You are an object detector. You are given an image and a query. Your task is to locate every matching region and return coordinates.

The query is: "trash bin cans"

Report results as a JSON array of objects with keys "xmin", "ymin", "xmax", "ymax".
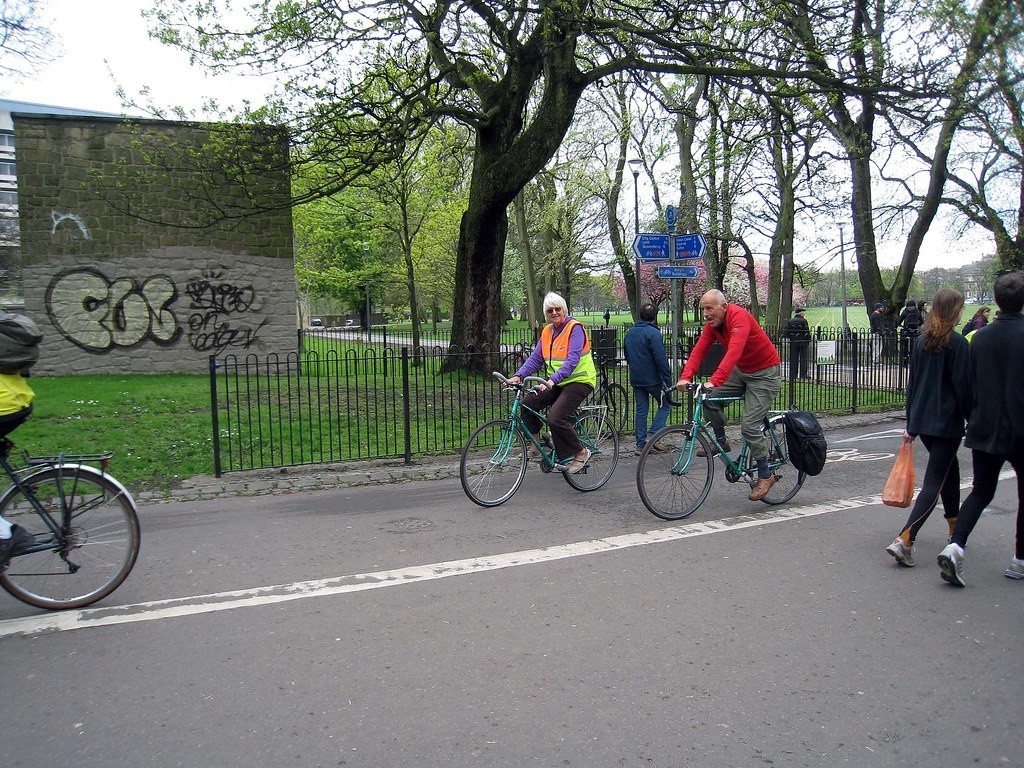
[
  {"xmin": 591, "ymin": 327, "xmax": 617, "ymax": 366},
  {"xmin": 700, "ymin": 341, "xmax": 724, "ymax": 378}
]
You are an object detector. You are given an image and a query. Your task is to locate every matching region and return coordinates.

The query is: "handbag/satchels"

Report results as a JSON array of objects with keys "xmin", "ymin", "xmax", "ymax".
[{"xmin": 883, "ymin": 441, "xmax": 914, "ymax": 507}]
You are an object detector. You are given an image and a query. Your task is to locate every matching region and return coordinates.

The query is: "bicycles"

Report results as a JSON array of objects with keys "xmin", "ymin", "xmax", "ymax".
[
  {"xmin": 636, "ymin": 381, "xmax": 810, "ymax": 523},
  {"xmin": 887, "ymin": 324, "xmax": 910, "ymax": 367},
  {"xmin": 0, "ymin": 434, "xmax": 140, "ymax": 611},
  {"xmin": 578, "ymin": 355, "xmax": 629, "ymax": 438},
  {"xmin": 460, "ymin": 370, "xmax": 622, "ymax": 509}
]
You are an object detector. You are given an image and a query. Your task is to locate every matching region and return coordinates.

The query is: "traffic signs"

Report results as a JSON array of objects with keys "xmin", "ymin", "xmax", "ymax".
[
  {"xmin": 631, "ymin": 233, "xmax": 671, "ymax": 260},
  {"xmin": 658, "ymin": 266, "xmax": 698, "ymax": 278},
  {"xmin": 673, "ymin": 234, "xmax": 708, "ymax": 262}
]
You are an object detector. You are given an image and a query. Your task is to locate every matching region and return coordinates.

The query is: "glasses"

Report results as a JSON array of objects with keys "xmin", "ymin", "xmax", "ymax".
[{"xmin": 545, "ymin": 307, "xmax": 563, "ymax": 314}]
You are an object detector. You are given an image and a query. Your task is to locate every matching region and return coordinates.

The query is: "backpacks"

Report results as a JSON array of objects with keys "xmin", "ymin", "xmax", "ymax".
[
  {"xmin": 0, "ymin": 309, "xmax": 44, "ymax": 374},
  {"xmin": 785, "ymin": 404, "xmax": 828, "ymax": 476},
  {"xmin": 962, "ymin": 317, "xmax": 978, "ymax": 336},
  {"xmin": 905, "ymin": 310, "xmax": 918, "ymax": 330}
]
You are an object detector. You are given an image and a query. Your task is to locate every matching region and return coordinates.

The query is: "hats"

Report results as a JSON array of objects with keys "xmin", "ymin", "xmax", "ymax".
[
  {"xmin": 876, "ymin": 302, "xmax": 883, "ymax": 308},
  {"xmin": 795, "ymin": 308, "xmax": 806, "ymax": 313}
]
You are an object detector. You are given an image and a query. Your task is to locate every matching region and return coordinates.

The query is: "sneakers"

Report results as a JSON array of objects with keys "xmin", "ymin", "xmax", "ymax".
[
  {"xmin": 653, "ymin": 441, "xmax": 667, "ymax": 451},
  {"xmin": 748, "ymin": 474, "xmax": 776, "ymax": 501},
  {"xmin": 887, "ymin": 538, "xmax": 914, "ymax": 566},
  {"xmin": 1004, "ymin": 555, "xmax": 1024, "ymax": 579},
  {"xmin": 938, "ymin": 541, "xmax": 968, "ymax": 588},
  {"xmin": 697, "ymin": 437, "xmax": 731, "ymax": 456},
  {"xmin": 635, "ymin": 446, "xmax": 654, "ymax": 456},
  {"xmin": 0, "ymin": 524, "xmax": 34, "ymax": 573}
]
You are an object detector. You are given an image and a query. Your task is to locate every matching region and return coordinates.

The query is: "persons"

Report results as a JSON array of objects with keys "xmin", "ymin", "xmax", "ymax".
[
  {"xmin": 887, "ymin": 289, "xmax": 971, "ymax": 566},
  {"xmin": 789, "ymin": 308, "xmax": 810, "ymax": 378},
  {"xmin": 503, "ymin": 292, "xmax": 596, "ymax": 472},
  {"xmin": 606, "ymin": 309, "xmax": 610, "ymax": 327},
  {"xmin": 871, "ymin": 303, "xmax": 884, "ymax": 366},
  {"xmin": 938, "ymin": 272, "xmax": 1024, "ymax": 587},
  {"xmin": 897, "ymin": 300, "xmax": 927, "ymax": 367},
  {"xmin": 624, "ymin": 305, "xmax": 675, "ymax": 456},
  {"xmin": 972, "ymin": 307, "xmax": 991, "ymax": 330},
  {"xmin": 677, "ymin": 289, "xmax": 780, "ymax": 501},
  {"xmin": 0, "ymin": 373, "xmax": 35, "ymax": 570}
]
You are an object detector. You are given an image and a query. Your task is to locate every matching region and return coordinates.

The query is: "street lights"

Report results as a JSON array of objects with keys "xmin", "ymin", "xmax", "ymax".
[
  {"xmin": 362, "ymin": 241, "xmax": 372, "ymax": 342},
  {"xmin": 834, "ymin": 220, "xmax": 850, "ymax": 328},
  {"xmin": 627, "ymin": 158, "xmax": 646, "ymax": 325}
]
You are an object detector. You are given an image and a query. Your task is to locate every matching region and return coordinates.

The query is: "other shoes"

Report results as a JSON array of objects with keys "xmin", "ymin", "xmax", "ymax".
[
  {"xmin": 801, "ymin": 375, "xmax": 812, "ymax": 378},
  {"xmin": 518, "ymin": 450, "xmax": 542, "ymax": 459},
  {"xmin": 569, "ymin": 448, "xmax": 592, "ymax": 474}
]
